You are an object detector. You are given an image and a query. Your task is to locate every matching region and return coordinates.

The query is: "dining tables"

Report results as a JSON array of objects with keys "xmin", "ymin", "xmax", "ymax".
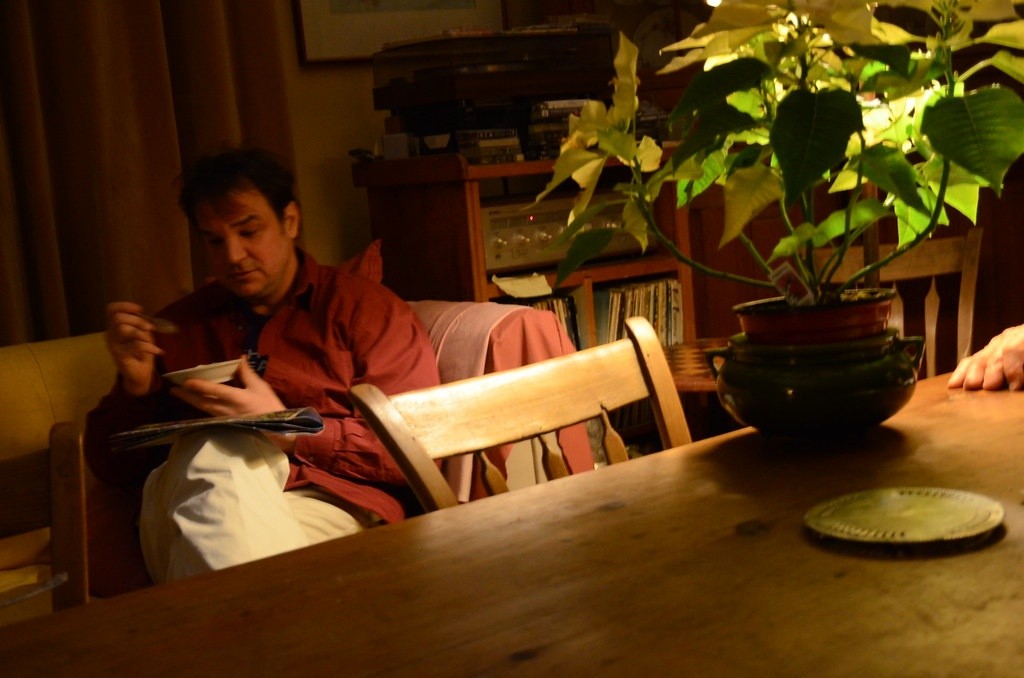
[{"xmin": 0, "ymin": 373, "xmax": 1022, "ymax": 678}]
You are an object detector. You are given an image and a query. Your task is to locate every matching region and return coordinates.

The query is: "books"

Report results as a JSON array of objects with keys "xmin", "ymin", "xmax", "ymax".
[
  {"xmin": 102, "ymin": 404, "xmax": 327, "ymax": 456},
  {"xmin": 548, "ymin": 12, "xmax": 617, "ymax": 32},
  {"xmin": 381, "ymin": 23, "xmax": 581, "ymax": 52},
  {"xmin": 490, "ymin": 274, "xmax": 690, "ymax": 473}
]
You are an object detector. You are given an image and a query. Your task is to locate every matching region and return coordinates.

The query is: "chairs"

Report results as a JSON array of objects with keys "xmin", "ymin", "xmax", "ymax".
[
  {"xmin": 796, "ymin": 226, "xmax": 982, "ymax": 379},
  {"xmin": 350, "ymin": 315, "xmax": 696, "ymax": 514},
  {"xmin": 45, "ymin": 300, "xmax": 594, "ymax": 610}
]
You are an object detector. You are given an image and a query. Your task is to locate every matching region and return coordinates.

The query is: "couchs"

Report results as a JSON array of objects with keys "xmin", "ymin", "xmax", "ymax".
[{"xmin": 0, "ymin": 330, "xmax": 120, "ymax": 631}]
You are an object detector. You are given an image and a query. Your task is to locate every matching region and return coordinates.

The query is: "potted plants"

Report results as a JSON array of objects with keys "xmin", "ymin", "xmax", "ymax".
[{"xmin": 521, "ymin": 1, "xmax": 1024, "ymax": 448}]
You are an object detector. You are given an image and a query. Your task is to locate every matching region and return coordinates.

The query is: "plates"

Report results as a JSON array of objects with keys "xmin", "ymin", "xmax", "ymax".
[{"xmin": 801, "ymin": 484, "xmax": 1005, "ymax": 546}]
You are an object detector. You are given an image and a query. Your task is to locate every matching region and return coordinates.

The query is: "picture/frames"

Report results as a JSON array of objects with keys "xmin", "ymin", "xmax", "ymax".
[{"xmin": 291, "ymin": 0, "xmax": 511, "ymax": 73}]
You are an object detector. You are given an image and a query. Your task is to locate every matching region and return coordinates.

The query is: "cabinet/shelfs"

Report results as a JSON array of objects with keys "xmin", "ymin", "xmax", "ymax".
[{"xmin": 351, "ymin": 140, "xmax": 705, "ymax": 442}]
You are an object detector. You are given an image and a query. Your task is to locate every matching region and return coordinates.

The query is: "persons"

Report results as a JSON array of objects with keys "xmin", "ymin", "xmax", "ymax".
[
  {"xmin": 82, "ymin": 141, "xmax": 448, "ymax": 591},
  {"xmin": 944, "ymin": 321, "xmax": 1024, "ymax": 399}
]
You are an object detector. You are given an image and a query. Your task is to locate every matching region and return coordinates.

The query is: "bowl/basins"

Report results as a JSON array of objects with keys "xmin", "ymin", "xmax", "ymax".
[{"xmin": 165, "ymin": 359, "xmax": 239, "ymax": 385}]
